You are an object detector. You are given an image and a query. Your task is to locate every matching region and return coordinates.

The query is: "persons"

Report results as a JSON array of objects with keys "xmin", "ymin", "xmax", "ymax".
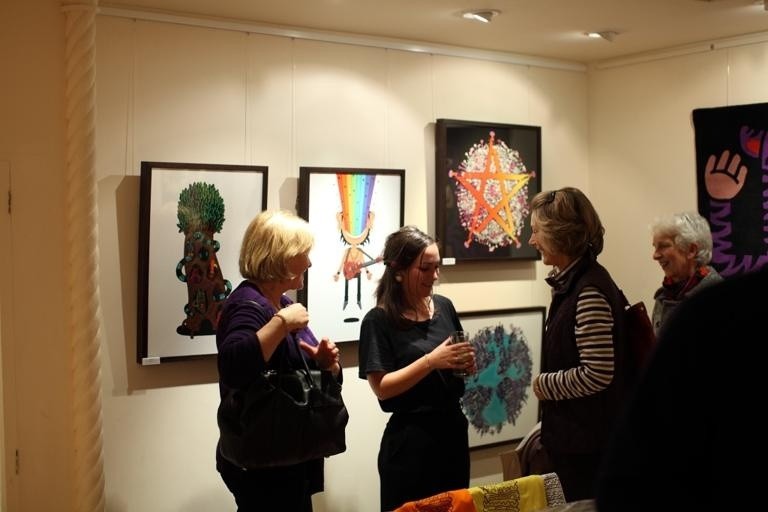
[
  {"xmin": 528, "ymin": 188, "xmax": 655, "ymax": 503},
  {"xmin": 652, "ymin": 211, "xmax": 724, "ymax": 336},
  {"xmin": 358, "ymin": 226, "xmax": 479, "ymax": 512},
  {"xmin": 215, "ymin": 206, "xmax": 349, "ymax": 512}
]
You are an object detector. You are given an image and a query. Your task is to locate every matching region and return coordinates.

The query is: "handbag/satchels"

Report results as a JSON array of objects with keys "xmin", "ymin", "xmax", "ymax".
[
  {"xmin": 219, "ymin": 367, "xmax": 348, "ymax": 468},
  {"xmin": 541, "ymin": 301, "xmax": 658, "ymax": 443}
]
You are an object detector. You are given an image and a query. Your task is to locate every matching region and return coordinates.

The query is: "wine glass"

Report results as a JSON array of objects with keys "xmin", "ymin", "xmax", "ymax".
[{"xmin": 449, "ymin": 331, "xmax": 470, "ymax": 376}]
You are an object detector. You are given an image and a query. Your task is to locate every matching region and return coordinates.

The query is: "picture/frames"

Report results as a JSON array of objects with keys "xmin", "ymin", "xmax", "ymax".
[
  {"xmin": 458, "ymin": 304, "xmax": 548, "ymax": 454},
  {"xmin": 434, "ymin": 117, "xmax": 543, "ymax": 264},
  {"xmin": 296, "ymin": 167, "xmax": 405, "ymax": 348},
  {"xmin": 134, "ymin": 161, "xmax": 269, "ymax": 365}
]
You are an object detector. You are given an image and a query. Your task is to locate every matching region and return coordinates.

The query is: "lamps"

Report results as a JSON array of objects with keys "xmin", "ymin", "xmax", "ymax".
[
  {"xmin": 590, "ymin": 32, "xmax": 614, "ymax": 46},
  {"xmin": 467, "ymin": 13, "xmax": 496, "ymax": 24}
]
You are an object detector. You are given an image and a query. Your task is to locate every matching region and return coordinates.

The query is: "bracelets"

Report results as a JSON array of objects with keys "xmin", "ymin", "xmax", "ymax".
[
  {"xmin": 273, "ymin": 314, "xmax": 288, "ymax": 333},
  {"xmin": 424, "ymin": 354, "xmax": 432, "ymax": 371}
]
